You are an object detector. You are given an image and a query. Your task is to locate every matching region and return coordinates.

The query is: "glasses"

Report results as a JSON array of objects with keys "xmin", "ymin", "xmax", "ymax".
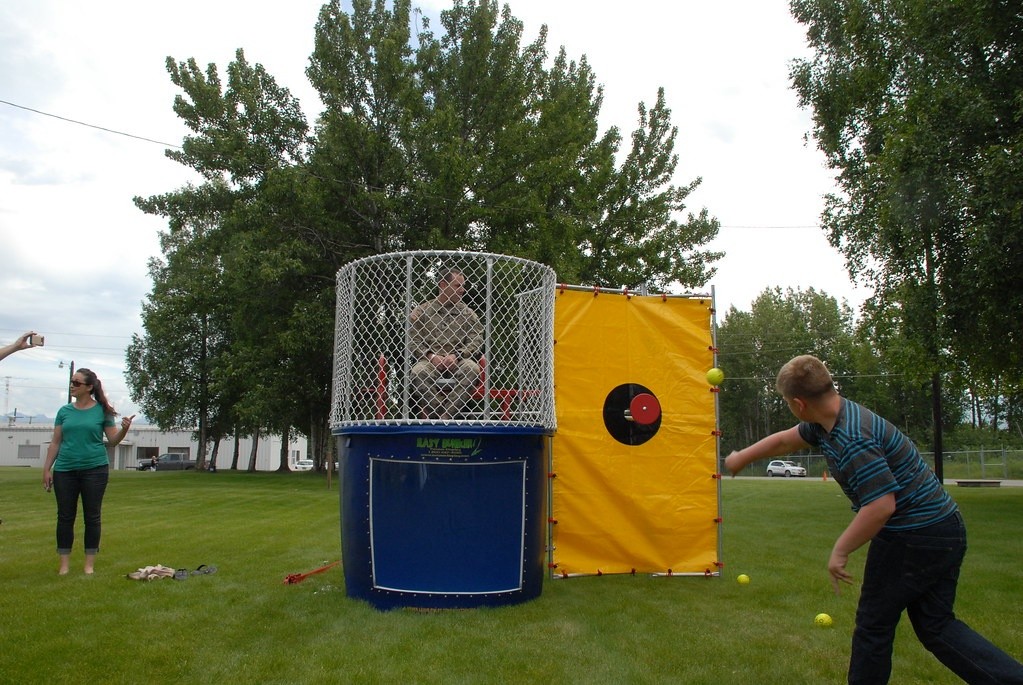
[{"xmin": 70, "ymin": 380, "xmax": 89, "ymax": 387}]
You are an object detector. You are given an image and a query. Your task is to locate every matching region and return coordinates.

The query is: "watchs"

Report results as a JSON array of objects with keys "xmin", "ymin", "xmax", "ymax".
[{"xmin": 455, "ymin": 353, "xmax": 463, "ymax": 360}]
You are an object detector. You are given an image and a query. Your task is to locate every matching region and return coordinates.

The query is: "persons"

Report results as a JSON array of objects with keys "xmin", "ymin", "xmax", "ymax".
[
  {"xmin": 725, "ymin": 355, "xmax": 1023, "ymax": 685},
  {"xmin": 0, "ymin": 330, "xmax": 37, "ymax": 361},
  {"xmin": 150, "ymin": 456, "xmax": 159, "ymax": 471},
  {"xmin": 408, "ymin": 268, "xmax": 485, "ymax": 419},
  {"xmin": 42, "ymin": 368, "xmax": 136, "ymax": 574}
]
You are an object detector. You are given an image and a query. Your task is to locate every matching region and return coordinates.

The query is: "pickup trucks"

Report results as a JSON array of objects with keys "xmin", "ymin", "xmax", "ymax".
[{"xmin": 139, "ymin": 452, "xmax": 213, "ymax": 471}]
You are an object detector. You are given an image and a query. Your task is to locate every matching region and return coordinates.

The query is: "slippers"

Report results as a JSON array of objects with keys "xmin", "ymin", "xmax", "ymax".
[
  {"xmin": 175, "ymin": 568, "xmax": 188, "ymax": 581},
  {"xmin": 191, "ymin": 564, "xmax": 217, "ymax": 576}
]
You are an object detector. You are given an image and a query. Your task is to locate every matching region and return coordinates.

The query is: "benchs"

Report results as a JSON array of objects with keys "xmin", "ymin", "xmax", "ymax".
[
  {"xmin": 359, "ymin": 353, "xmax": 543, "ymax": 420},
  {"xmin": 956, "ymin": 481, "xmax": 1001, "ymax": 487}
]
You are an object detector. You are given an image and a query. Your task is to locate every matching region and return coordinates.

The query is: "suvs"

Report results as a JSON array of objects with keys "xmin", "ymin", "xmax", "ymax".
[{"xmin": 766, "ymin": 459, "xmax": 807, "ymax": 477}]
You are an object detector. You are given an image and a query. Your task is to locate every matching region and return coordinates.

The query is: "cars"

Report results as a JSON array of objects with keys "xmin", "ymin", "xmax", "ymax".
[{"xmin": 294, "ymin": 460, "xmax": 314, "ymax": 472}]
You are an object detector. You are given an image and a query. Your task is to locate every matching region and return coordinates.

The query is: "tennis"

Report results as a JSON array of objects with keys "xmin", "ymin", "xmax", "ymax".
[
  {"xmin": 814, "ymin": 613, "xmax": 833, "ymax": 626},
  {"xmin": 737, "ymin": 573, "xmax": 750, "ymax": 585},
  {"xmin": 706, "ymin": 368, "xmax": 724, "ymax": 386}
]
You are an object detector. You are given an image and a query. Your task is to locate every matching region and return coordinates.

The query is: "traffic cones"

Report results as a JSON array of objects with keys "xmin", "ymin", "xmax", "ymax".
[{"xmin": 822, "ymin": 471, "xmax": 827, "ymax": 480}]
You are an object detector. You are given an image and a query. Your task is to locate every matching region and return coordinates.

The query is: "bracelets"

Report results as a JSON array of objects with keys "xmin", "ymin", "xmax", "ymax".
[{"xmin": 431, "ymin": 354, "xmax": 435, "ymax": 358}]
